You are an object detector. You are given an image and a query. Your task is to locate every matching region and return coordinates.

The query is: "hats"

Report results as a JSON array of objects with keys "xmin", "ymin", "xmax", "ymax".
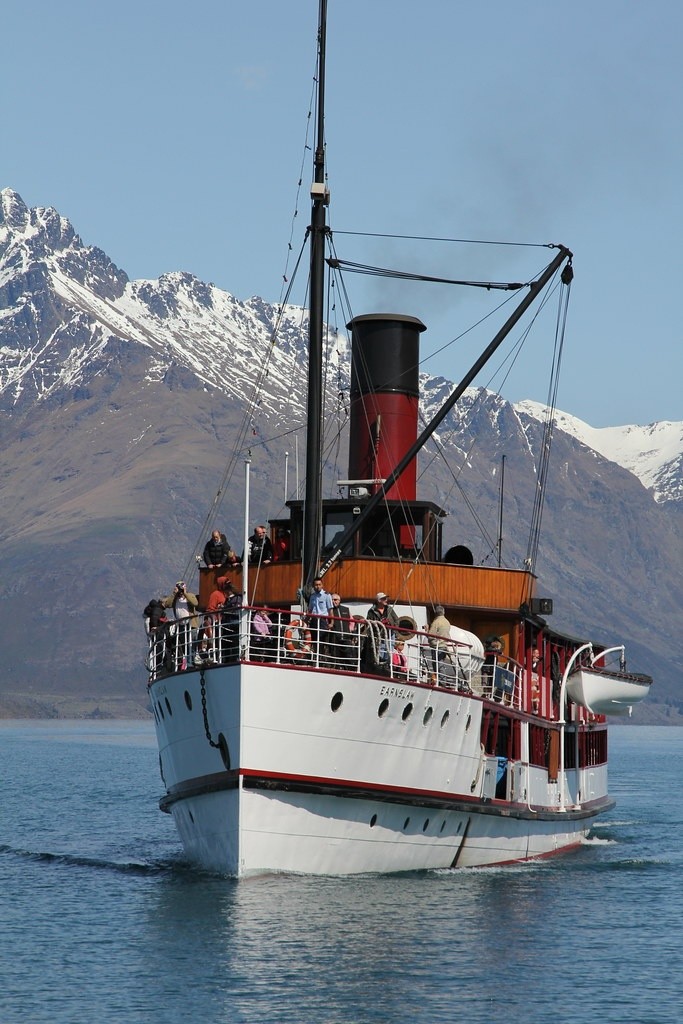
[{"xmin": 376, "ymin": 592, "xmax": 389, "ymax": 600}]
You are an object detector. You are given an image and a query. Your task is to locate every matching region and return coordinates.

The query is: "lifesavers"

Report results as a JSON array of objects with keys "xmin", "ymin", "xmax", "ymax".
[
  {"xmin": 285, "ymin": 619, "xmax": 312, "ymax": 658},
  {"xmin": 197, "ymin": 621, "xmax": 213, "ymax": 660}
]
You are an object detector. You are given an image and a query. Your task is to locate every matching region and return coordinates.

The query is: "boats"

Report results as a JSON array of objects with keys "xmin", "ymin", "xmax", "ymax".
[{"xmin": 567, "ymin": 662, "xmax": 654, "ymax": 714}]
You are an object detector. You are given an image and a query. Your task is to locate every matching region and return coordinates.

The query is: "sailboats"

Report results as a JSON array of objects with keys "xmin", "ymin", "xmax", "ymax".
[{"xmin": 144, "ymin": 0, "xmax": 624, "ymax": 881}]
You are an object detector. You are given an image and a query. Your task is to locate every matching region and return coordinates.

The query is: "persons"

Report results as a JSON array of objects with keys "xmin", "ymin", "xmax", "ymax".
[
  {"xmin": 531, "ymin": 647, "xmax": 543, "ymax": 674},
  {"xmin": 203, "ymin": 525, "xmax": 274, "ymax": 569},
  {"xmin": 254, "ymin": 604, "xmax": 272, "ymax": 662},
  {"xmin": 428, "ymin": 606, "xmax": 451, "ymax": 687},
  {"xmin": 366, "ymin": 592, "xmax": 415, "ymax": 680},
  {"xmin": 142, "ymin": 576, "xmax": 234, "ymax": 672},
  {"xmin": 306, "ymin": 577, "xmax": 356, "ymax": 668}
]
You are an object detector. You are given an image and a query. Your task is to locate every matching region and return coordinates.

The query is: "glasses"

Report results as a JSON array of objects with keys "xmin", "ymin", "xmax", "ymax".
[
  {"xmin": 332, "ymin": 599, "xmax": 340, "ymax": 601},
  {"xmin": 380, "ymin": 597, "xmax": 386, "ymax": 601}
]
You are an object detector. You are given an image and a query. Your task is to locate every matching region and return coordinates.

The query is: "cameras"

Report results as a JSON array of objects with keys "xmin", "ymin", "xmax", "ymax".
[{"xmin": 177, "ymin": 585, "xmax": 184, "ymax": 593}]
